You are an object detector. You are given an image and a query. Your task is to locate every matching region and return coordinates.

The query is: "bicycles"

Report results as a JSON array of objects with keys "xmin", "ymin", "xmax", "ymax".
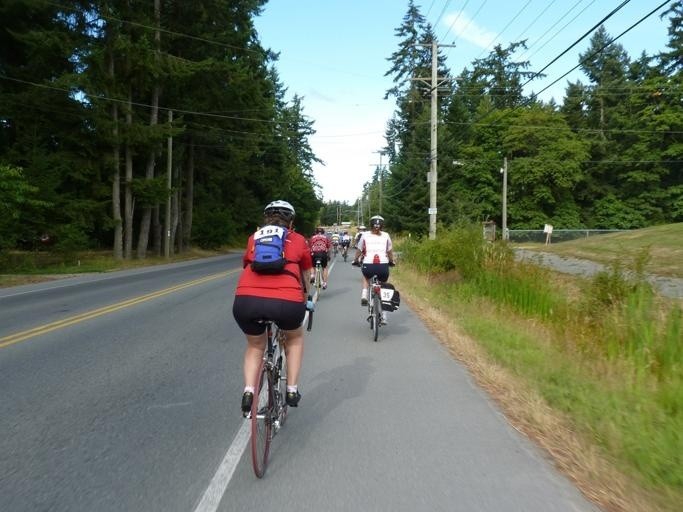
[
  {"xmin": 242, "ymin": 320, "xmax": 297, "ymax": 479},
  {"xmin": 351, "ymin": 262, "xmax": 397, "ymax": 341},
  {"xmin": 311, "ymin": 243, "xmax": 349, "ymax": 304}
]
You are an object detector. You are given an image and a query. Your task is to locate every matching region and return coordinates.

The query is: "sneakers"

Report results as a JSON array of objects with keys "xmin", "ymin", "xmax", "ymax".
[
  {"xmin": 285, "ymin": 390, "xmax": 302, "ymax": 406},
  {"xmin": 322, "ymin": 284, "xmax": 327, "ymax": 289},
  {"xmin": 381, "ymin": 318, "xmax": 388, "ymax": 326},
  {"xmin": 241, "ymin": 392, "xmax": 253, "ymax": 413},
  {"xmin": 361, "ymin": 294, "xmax": 368, "ymax": 306},
  {"xmin": 310, "ymin": 275, "xmax": 315, "ymax": 283}
]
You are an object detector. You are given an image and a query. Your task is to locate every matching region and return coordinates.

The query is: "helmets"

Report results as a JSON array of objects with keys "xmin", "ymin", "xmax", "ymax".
[
  {"xmin": 359, "ymin": 226, "xmax": 367, "ymax": 230},
  {"xmin": 370, "ymin": 215, "xmax": 384, "ymax": 222},
  {"xmin": 263, "ymin": 200, "xmax": 296, "ymax": 217}
]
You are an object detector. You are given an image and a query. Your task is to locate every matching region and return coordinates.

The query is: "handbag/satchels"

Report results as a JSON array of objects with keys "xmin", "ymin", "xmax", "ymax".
[{"xmin": 380, "ymin": 282, "xmax": 400, "ymax": 312}]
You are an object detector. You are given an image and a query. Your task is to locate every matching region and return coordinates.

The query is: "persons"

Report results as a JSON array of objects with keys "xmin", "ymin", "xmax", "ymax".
[
  {"xmin": 306, "ymin": 227, "xmax": 330, "ymax": 289},
  {"xmin": 325, "ymin": 221, "xmax": 366, "ymax": 266},
  {"xmin": 231, "ymin": 198, "xmax": 314, "ymax": 412},
  {"xmin": 352, "ymin": 213, "xmax": 396, "ymax": 326}
]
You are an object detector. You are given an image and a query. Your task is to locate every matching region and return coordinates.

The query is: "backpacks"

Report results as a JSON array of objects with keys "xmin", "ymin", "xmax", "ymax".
[
  {"xmin": 356, "ymin": 234, "xmax": 362, "ymax": 247},
  {"xmin": 252, "ymin": 224, "xmax": 286, "ymax": 274},
  {"xmin": 332, "ymin": 236, "xmax": 339, "ymax": 246},
  {"xmin": 344, "ymin": 239, "xmax": 350, "ymax": 244},
  {"xmin": 311, "ymin": 237, "xmax": 328, "ymax": 257}
]
